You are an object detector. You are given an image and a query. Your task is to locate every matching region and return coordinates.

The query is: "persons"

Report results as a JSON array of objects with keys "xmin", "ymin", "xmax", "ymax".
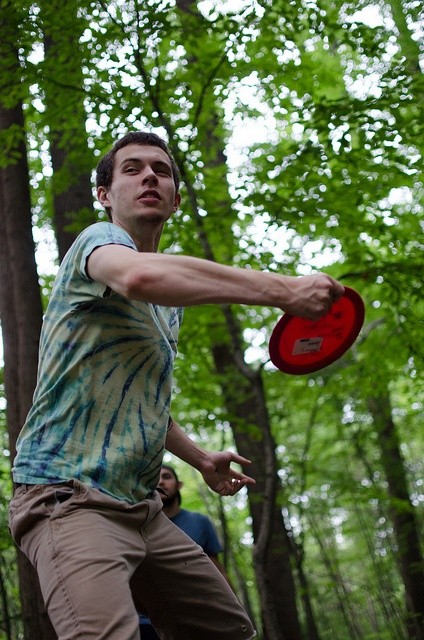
[
  {"xmin": 128, "ymin": 465, "xmax": 237, "ymax": 633},
  {"xmin": 6, "ymin": 133, "xmax": 345, "ymax": 640}
]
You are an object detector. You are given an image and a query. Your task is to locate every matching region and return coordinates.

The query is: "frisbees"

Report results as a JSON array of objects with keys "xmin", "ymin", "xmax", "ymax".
[{"xmin": 269, "ymin": 285, "xmax": 365, "ymax": 374}]
